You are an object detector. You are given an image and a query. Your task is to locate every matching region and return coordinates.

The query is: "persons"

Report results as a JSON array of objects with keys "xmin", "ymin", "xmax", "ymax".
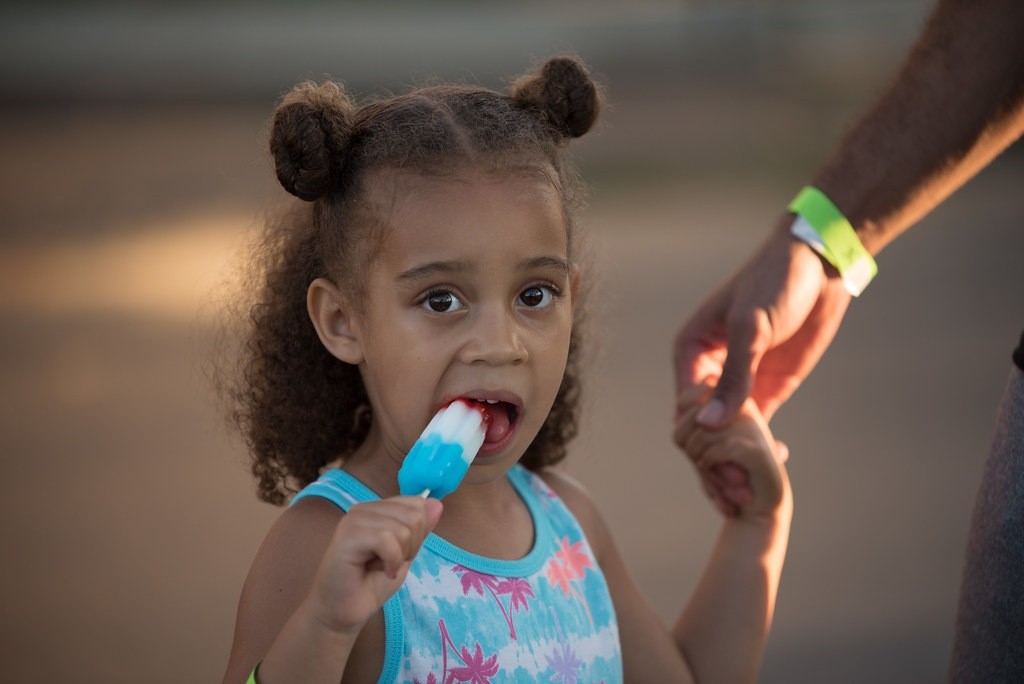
[
  {"xmin": 224, "ymin": 55, "xmax": 793, "ymax": 684},
  {"xmin": 668, "ymin": 0, "xmax": 1024, "ymax": 684}
]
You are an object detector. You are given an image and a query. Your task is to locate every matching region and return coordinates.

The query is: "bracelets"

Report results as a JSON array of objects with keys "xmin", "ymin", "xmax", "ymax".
[
  {"xmin": 246, "ymin": 659, "xmax": 263, "ymax": 684},
  {"xmin": 785, "ymin": 184, "xmax": 878, "ymax": 297}
]
